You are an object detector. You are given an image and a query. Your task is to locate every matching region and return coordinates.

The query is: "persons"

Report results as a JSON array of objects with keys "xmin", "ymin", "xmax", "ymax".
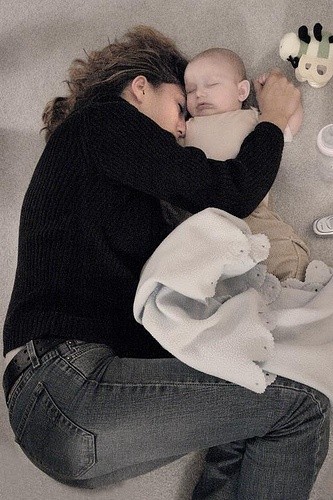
[
  {"xmin": 166, "ymin": 48, "xmax": 309, "ymax": 282},
  {"xmin": 2, "ymin": 25, "xmax": 332, "ymax": 499}
]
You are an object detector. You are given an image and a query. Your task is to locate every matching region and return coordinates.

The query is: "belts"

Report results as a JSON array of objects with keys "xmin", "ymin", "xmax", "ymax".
[{"xmin": 3, "ymin": 338, "xmax": 68, "ymax": 406}]
